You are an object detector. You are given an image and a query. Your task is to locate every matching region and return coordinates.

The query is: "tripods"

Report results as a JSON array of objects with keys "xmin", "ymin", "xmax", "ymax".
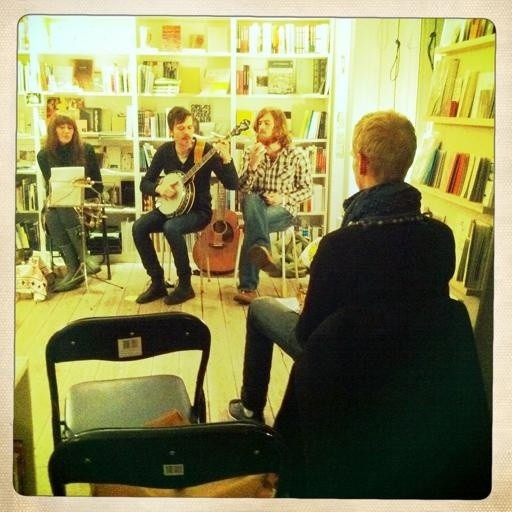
[{"xmin": 51, "ymin": 187, "xmax": 123, "ymax": 311}]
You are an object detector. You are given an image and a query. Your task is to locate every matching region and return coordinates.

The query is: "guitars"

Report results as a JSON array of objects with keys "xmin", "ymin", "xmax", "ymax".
[{"xmin": 192, "ymin": 140, "xmax": 239, "ymax": 274}]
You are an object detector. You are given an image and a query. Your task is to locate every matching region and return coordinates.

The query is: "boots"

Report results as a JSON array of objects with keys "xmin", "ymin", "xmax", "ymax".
[
  {"xmin": 163, "ymin": 268, "xmax": 194, "ymax": 305},
  {"xmin": 68, "ymin": 225, "xmax": 101, "ymax": 274},
  {"xmin": 136, "ymin": 267, "xmax": 168, "ymax": 303},
  {"xmin": 54, "ymin": 243, "xmax": 85, "ymax": 292}
]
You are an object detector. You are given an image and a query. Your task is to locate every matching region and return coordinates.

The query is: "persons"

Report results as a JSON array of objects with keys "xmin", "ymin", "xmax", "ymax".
[
  {"xmin": 228, "ymin": 111, "xmax": 456, "ymax": 426},
  {"xmin": 131, "ymin": 106, "xmax": 238, "ymax": 305},
  {"xmin": 37, "ymin": 110, "xmax": 104, "ymax": 292},
  {"xmin": 233, "ymin": 106, "xmax": 313, "ymax": 305}
]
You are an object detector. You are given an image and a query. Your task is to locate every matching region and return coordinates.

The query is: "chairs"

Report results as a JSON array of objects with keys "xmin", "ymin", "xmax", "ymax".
[
  {"xmin": 45, "ymin": 311, "xmax": 211, "ymax": 449},
  {"xmin": 48, "ymin": 421, "xmax": 286, "ymax": 496}
]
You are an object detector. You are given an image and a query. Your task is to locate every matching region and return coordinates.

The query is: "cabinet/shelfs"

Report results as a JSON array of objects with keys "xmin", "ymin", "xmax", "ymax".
[
  {"xmin": 17, "ymin": 15, "xmax": 336, "ymax": 266},
  {"xmin": 408, "ymin": 16, "xmax": 494, "ymax": 347}
]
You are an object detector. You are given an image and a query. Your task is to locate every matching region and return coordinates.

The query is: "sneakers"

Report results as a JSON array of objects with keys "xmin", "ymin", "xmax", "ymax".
[
  {"xmin": 247, "ymin": 245, "xmax": 276, "ymax": 272},
  {"xmin": 234, "ymin": 289, "xmax": 257, "ymax": 304},
  {"xmin": 229, "ymin": 399, "xmax": 266, "ymax": 425}
]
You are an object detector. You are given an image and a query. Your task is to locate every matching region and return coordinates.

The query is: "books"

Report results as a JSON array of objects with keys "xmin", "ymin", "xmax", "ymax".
[
  {"xmin": 420, "ymin": 18, "xmax": 496, "ymax": 297},
  {"xmin": 16, "ymin": 22, "xmax": 133, "ymax": 253},
  {"xmin": 135, "ymin": 23, "xmax": 231, "ymax": 253},
  {"xmin": 234, "ymin": 22, "xmax": 328, "ymax": 242}
]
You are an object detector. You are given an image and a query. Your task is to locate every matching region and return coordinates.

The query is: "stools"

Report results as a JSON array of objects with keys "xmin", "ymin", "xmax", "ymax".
[{"xmin": 268, "ymin": 227, "xmax": 304, "ymax": 307}]
